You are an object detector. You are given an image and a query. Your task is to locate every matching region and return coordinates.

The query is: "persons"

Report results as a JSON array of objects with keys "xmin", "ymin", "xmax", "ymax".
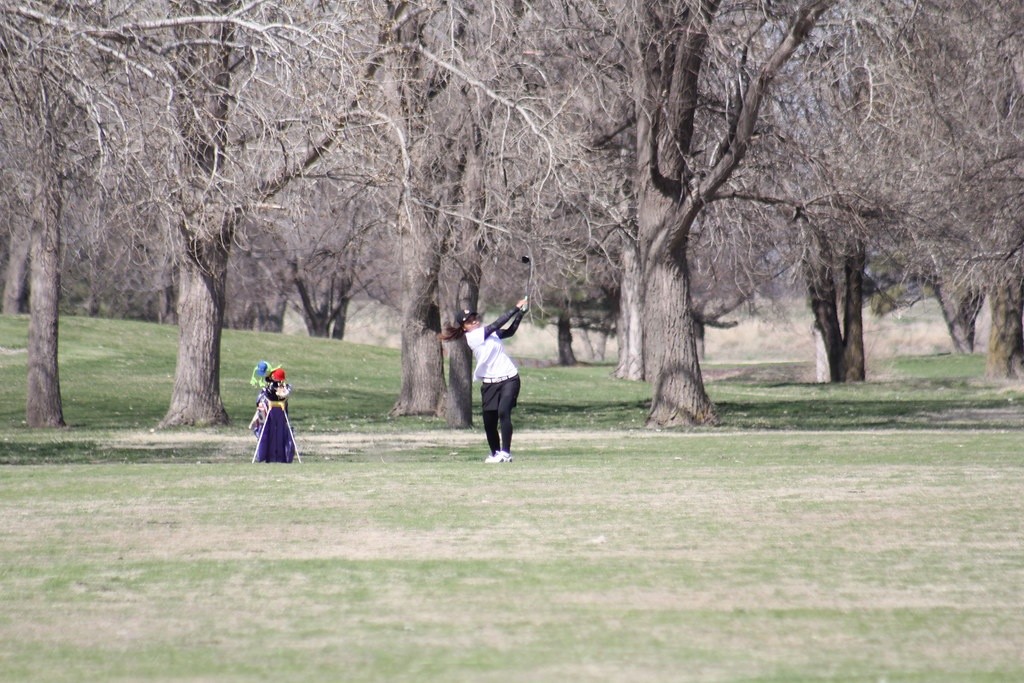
[{"xmin": 437, "ymin": 295, "xmax": 529, "ymax": 464}]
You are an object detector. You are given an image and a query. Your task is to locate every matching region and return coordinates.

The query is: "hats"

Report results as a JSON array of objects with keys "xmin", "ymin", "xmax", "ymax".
[{"xmin": 457, "ymin": 308, "xmax": 478, "ymax": 323}]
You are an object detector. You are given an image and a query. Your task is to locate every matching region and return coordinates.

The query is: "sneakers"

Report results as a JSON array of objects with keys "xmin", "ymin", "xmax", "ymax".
[
  {"xmin": 493, "ymin": 451, "xmax": 512, "ymax": 462},
  {"xmin": 485, "ymin": 451, "xmax": 500, "ymax": 463}
]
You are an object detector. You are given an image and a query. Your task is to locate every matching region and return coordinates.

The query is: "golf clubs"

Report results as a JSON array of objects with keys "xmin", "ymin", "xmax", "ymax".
[
  {"xmin": 262, "ymin": 381, "xmax": 292, "ymax": 401},
  {"xmin": 522, "ymin": 255, "xmax": 533, "ymax": 300}
]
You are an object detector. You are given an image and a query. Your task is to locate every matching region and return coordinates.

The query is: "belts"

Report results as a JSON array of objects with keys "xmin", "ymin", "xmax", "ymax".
[{"xmin": 482, "ymin": 371, "xmax": 518, "ymax": 383}]
